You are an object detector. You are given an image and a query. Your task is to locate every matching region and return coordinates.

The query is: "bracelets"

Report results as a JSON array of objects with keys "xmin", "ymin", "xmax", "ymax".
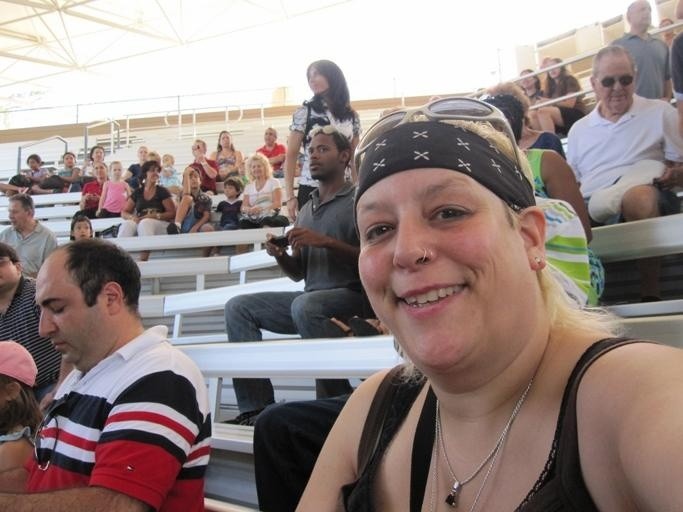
[{"xmin": 285, "ymin": 197, "xmax": 301, "ymax": 202}]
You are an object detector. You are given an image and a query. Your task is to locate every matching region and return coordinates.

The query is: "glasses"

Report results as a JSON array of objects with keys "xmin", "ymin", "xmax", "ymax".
[
  {"xmin": 601, "ymin": 75, "xmax": 634, "ymax": 87},
  {"xmin": 354, "ymin": 97, "xmax": 522, "ymax": 173},
  {"xmin": 308, "ymin": 125, "xmax": 339, "ymax": 137}
]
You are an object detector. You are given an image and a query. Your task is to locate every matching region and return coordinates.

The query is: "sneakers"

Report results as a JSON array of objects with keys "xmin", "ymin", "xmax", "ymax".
[{"xmin": 218, "ymin": 409, "xmax": 261, "ymax": 426}]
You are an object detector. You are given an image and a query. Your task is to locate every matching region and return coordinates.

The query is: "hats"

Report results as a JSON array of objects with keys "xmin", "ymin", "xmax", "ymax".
[{"xmin": 0, "ymin": 338, "xmax": 39, "ymax": 388}]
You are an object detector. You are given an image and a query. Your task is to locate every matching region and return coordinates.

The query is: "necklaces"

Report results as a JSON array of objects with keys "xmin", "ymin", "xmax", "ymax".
[{"xmin": 429, "ymin": 383, "xmax": 534, "ymax": 511}]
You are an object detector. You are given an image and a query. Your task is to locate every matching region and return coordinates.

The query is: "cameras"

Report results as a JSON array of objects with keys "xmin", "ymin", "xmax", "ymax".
[{"xmin": 269, "ymin": 236, "xmax": 290, "ymax": 248}]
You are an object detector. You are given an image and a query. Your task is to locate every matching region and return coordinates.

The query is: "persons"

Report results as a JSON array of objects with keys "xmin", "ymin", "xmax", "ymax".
[
  {"xmin": 0, "ymin": 245, "xmax": 73, "ymax": 412},
  {"xmin": 0, "ymin": 195, "xmax": 60, "ymax": 276},
  {"xmin": 283, "ymin": 98, "xmax": 683, "ymax": 512},
  {"xmin": 484, "ymin": 0, "xmax": 683, "ymax": 302},
  {"xmin": 1, "ymin": 118, "xmax": 285, "ymax": 237},
  {"xmin": 220, "ymin": 124, "xmax": 375, "ymax": 425},
  {"xmin": 253, "ymin": 391, "xmax": 354, "ymax": 512},
  {"xmin": 1, "ymin": 341, "xmax": 45, "ymax": 470},
  {"xmin": 321, "ymin": 315, "xmax": 389, "ymax": 339},
  {"xmin": 286, "ymin": 60, "xmax": 361, "ymax": 220},
  {"xmin": 0, "ymin": 239, "xmax": 211, "ymax": 512}
]
